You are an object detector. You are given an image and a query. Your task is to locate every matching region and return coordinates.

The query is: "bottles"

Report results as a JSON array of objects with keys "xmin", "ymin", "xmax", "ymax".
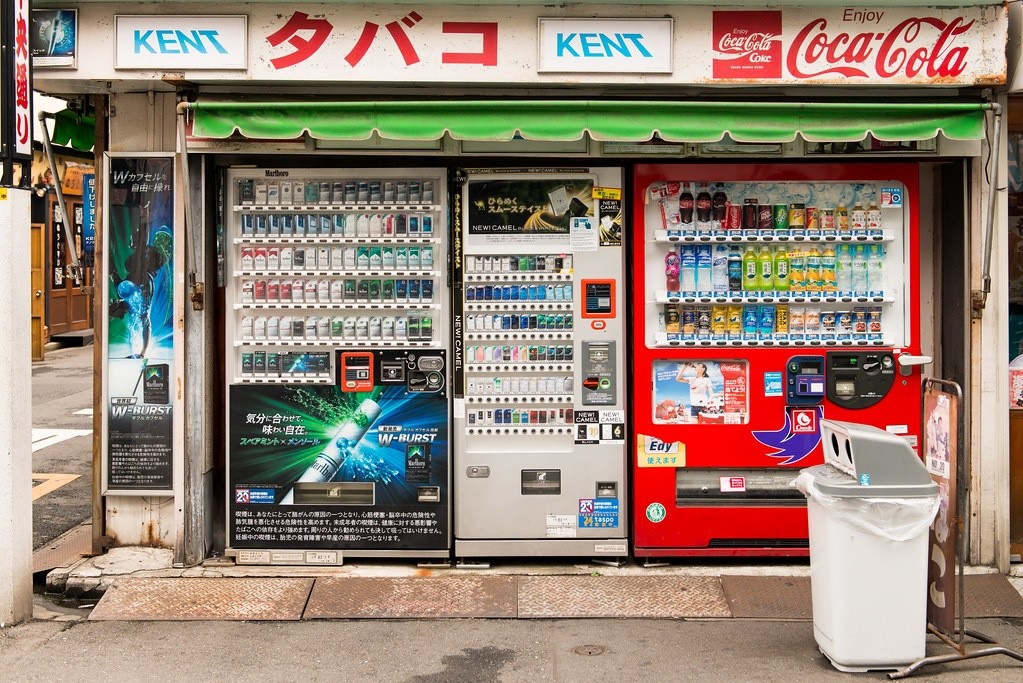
[
  {"xmin": 684, "ymin": 362, "xmax": 696, "ymax": 369},
  {"xmin": 699, "ymin": 398, "xmax": 725, "ymax": 414},
  {"xmin": 867, "ymin": 202, "xmax": 882, "ymax": 230},
  {"xmin": 680, "ymin": 181, "xmax": 729, "ymax": 230},
  {"xmin": 666, "ymin": 243, "xmax": 888, "ymax": 299},
  {"xmin": 850, "ymin": 201, "xmax": 867, "ymax": 230}
]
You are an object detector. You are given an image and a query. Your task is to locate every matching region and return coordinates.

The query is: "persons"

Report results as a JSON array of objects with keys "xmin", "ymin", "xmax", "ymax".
[
  {"xmin": 109, "ymin": 197, "xmax": 171, "ymax": 360},
  {"xmin": 30, "ymin": 168, "xmax": 53, "ymax": 224},
  {"xmin": 677, "ymin": 362, "xmax": 716, "ymax": 418}
]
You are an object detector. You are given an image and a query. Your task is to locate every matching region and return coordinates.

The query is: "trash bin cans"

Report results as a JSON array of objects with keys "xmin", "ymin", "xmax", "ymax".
[{"xmin": 789, "ymin": 418, "xmax": 942, "ymax": 676}]
